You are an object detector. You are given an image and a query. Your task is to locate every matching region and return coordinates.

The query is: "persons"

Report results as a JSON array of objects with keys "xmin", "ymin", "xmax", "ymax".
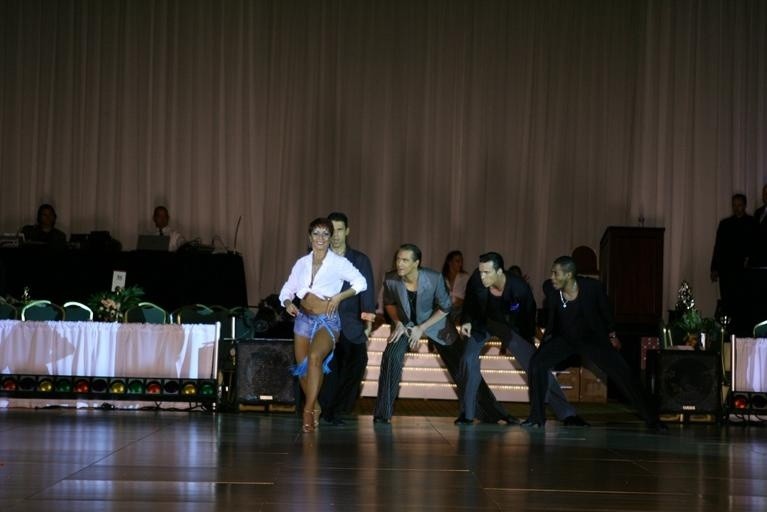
[
  {"xmin": 307, "ymin": 211, "xmax": 377, "ymax": 425},
  {"xmin": 755, "ymin": 184, "xmax": 767, "ymax": 225},
  {"xmin": 510, "ymin": 263, "xmax": 530, "ymax": 281},
  {"xmin": 152, "ymin": 205, "xmax": 188, "ymax": 252},
  {"xmin": 710, "ymin": 193, "xmax": 757, "ymax": 298},
  {"xmin": 374, "ymin": 275, "xmax": 386, "ymax": 330},
  {"xmin": 454, "ymin": 248, "xmax": 591, "ymax": 427},
  {"xmin": 36, "ymin": 204, "xmax": 66, "ymax": 250},
  {"xmin": 374, "ymin": 242, "xmax": 520, "ymax": 426},
  {"xmin": 520, "ymin": 255, "xmax": 663, "ymax": 433},
  {"xmin": 429, "ymin": 250, "xmax": 470, "ymax": 353},
  {"xmin": 279, "ymin": 217, "xmax": 369, "ymax": 433}
]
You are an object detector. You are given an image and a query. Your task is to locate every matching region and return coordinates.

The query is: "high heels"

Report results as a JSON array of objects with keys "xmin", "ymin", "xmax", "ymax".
[{"xmin": 302, "ymin": 409, "xmax": 321, "ymax": 433}]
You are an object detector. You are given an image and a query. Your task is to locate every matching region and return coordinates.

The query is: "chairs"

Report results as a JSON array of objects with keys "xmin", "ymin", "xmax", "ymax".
[{"xmin": 1, "ymin": 298, "xmax": 256, "ymax": 402}]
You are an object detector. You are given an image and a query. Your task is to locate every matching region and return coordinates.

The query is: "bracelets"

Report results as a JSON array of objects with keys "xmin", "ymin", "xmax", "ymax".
[{"xmin": 609, "ymin": 334, "xmax": 618, "ymax": 339}]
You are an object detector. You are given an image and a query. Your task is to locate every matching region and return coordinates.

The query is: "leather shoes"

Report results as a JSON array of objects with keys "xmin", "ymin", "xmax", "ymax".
[
  {"xmin": 649, "ymin": 423, "xmax": 670, "ymax": 435},
  {"xmin": 454, "ymin": 416, "xmax": 590, "ymax": 428},
  {"xmin": 374, "ymin": 416, "xmax": 387, "ymax": 423}
]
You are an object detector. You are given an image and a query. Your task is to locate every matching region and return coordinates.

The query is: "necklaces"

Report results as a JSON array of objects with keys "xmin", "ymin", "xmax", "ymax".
[{"xmin": 560, "ymin": 280, "xmax": 577, "ymax": 309}]
